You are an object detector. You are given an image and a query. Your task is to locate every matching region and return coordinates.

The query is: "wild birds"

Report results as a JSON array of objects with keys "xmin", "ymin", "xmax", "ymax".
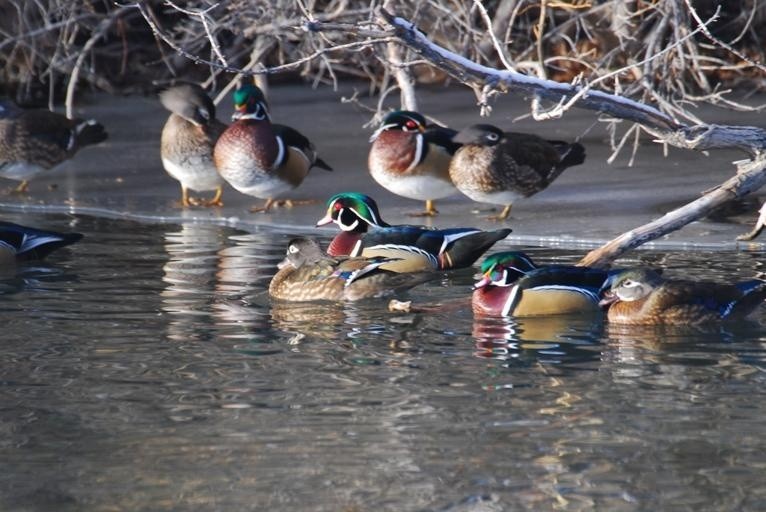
[
  {"xmin": 267, "ymin": 235, "xmax": 452, "ymax": 301},
  {"xmin": 449, "ymin": 124, "xmax": 586, "ymax": 219},
  {"xmin": 0, "ymin": 92, "xmax": 108, "ymax": 193},
  {"xmin": 156, "ymin": 81, "xmax": 228, "ymax": 207},
  {"xmin": 470, "ymin": 250, "xmax": 665, "ymax": 317},
  {"xmin": 598, "ymin": 266, "xmax": 766, "ymax": 326},
  {"xmin": 0, "ymin": 220, "xmax": 83, "ymax": 260},
  {"xmin": 315, "ymin": 192, "xmax": 513, "ymax": 271},
  {"xmin": 212, "ymin": 84, "xmax": 334, "ymax": 213},
  {"xmin": 367, "ymin": 111, "xmax": 463, "ymax": 216}
]
